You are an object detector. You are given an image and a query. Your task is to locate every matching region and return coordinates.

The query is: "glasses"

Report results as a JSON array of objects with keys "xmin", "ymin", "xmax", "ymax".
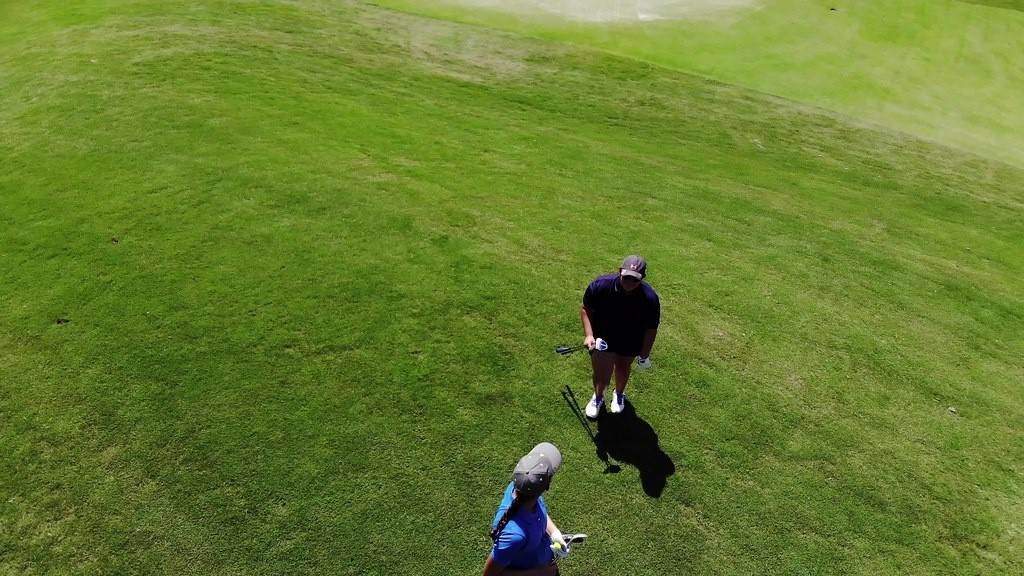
[{"xmin": 621, "ymin": 275, "xmax": 640, "ymax": 282}]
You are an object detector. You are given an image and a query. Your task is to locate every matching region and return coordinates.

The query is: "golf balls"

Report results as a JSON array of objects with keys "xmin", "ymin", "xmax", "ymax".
[{"xmin": 553, "ymin": 542, "xmax": 561, "ymax": 550}]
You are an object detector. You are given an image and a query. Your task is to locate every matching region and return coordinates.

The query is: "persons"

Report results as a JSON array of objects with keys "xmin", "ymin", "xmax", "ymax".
[
  {"xmin": 482, "ymin": 442, "xmax": 571, "ymax": 576},
  {"xmin": 580, "ymin": 255, "xmax": 660, "ymax": 420}
]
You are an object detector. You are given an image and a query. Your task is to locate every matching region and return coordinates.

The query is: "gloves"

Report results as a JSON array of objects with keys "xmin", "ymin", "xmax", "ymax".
[{"xmin": 549, "ymin": 529, "xmax": 569, "ymax": 558}]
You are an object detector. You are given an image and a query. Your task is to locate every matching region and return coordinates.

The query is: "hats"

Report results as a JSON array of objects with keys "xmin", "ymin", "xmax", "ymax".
[
  {"xmin": 619, "ymin": 255, "xmax": 647, "ymax": 279},
  {"xmin": 512, "ymin": 442, "xmax": 562, "ymax": 496}
]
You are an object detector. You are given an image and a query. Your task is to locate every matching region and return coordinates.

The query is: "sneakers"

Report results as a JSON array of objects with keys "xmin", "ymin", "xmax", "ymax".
[
  {"xmin": 611, "ymin": 389, "xmax": 624, "ymax": 414},
  {"xmin": 586, "ymin": 393, "xmax": 605, "ymax": 418}
]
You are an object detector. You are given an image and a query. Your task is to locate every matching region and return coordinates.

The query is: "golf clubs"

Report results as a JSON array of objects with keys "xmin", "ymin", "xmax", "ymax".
[
  {"xmin": 550, "ymin": 533, "xmax": 588, "ymax": 566},
  {"xmin": 556, "ymin": 337, "xmax": 608, "ymax": 356}
]
